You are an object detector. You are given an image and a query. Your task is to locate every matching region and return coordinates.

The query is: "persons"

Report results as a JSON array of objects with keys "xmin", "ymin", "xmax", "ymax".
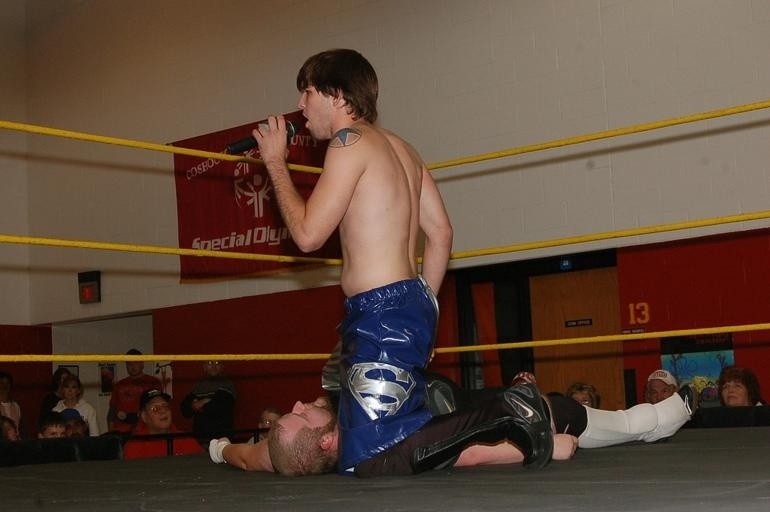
[
  {"xmin": 567, "ymin": 383, "xmax": 599, "ymax": 409},
  {"xmin": 647, "ymin": 368, "xmax": 678, "ymax": 403},
  {"xmin": 719, "ymin": 363, "xmax": 763, "ymax": 408},
  {"xmin": 253, "ymin": 48, "xmax": 553, "ymax": 479},
  {"xmin": 0, "ymin": 349, "xmax": 282, "ymax": 460},
  {"xmin": 209, "ymin": 371, "xmax": 700, "ymax": 477}
]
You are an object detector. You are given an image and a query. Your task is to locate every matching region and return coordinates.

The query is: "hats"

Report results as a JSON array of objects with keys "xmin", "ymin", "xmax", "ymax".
[
  {"xmin": 140, "ymin": 389, "xmax": 170, "ymax": 407},
  {"xmin": 61, "ymin": 408, "xmax": 81, "ymax": 423},
  {"xmin": 647, "ymin": 370, "xmax": 678, "ymax": 389}
]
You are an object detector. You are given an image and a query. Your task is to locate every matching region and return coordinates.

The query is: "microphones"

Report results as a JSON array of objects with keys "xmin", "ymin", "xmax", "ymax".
[{"xmin": 227, "ymin": 120, "xmax": 299, "ymax": 155}]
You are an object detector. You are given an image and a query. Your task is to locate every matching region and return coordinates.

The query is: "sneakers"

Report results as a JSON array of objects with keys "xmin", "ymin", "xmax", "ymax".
[
  {"xmin": 507, "ymin": 382, "xmax": 553, "ymax": 469},
  {"xmin": 678, "ymin": 383, "xmax": 697, "ymax": 417}
]
